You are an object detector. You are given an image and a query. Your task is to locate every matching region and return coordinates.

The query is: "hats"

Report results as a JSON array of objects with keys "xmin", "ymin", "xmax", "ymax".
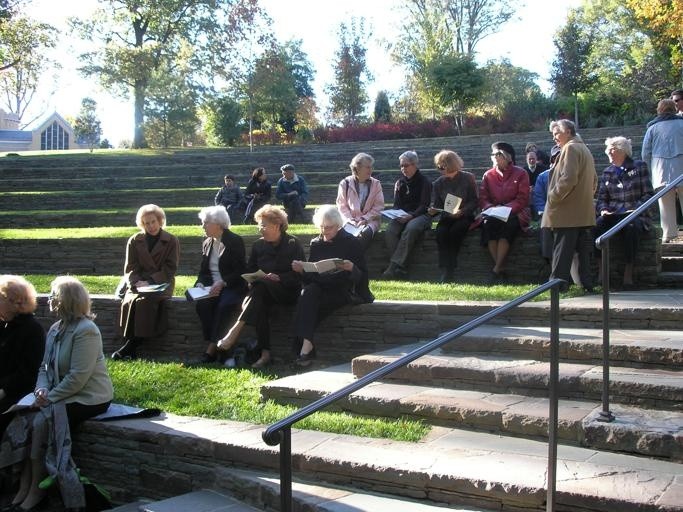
[
  {"xmin": 281, "ymin": 163, "xmax": 295, "ymax": 171},
  {"xmin": 490, "ymin": 141, "xmax": 517, "ymax": 166},
  {"xmin": 550, "ymin": 143, "xmax": 559, "ymax": 163}
]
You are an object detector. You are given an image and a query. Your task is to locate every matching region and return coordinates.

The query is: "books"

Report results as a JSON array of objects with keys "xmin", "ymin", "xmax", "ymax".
[
  {"xmin": 480, "ymin": 205, "xmax": 513, "ymax": 222},
  {"xmin": 187, "ymin": 286, "xmax": 212, "ymax": 301},
  {"xmin": 302, "ymin": 257, "xmax": 344, "ymax": 278}
]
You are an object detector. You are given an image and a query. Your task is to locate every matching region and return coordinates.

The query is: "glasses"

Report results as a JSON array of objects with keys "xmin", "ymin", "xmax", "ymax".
[
  {"xmin": 399, "ymin": 163, "xmax": 414, "ymax": 168},
  {"xmin": 674, "ymin": 98, "xmax": 682, "ymax": 102},
  {"xmin": 437, "ymin": 166, "xmax": 447, "ymax": 171}
]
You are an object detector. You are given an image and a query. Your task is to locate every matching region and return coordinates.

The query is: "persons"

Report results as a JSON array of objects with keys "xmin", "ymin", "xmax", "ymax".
[
  {"xmin": 240, "ymin": 167, "xmax": 272, "ymax": 225},
  {"xmin": 335, "ymin": 152, "xmax": 384, "ymax": 247},
  {"xmin": 0, "ymin": 273, "xmax": 46, "ymax": 440},
  {"xmin": 274, "ymin": 163, "xmax": 309, "ymax": 222},
  {"xmin": 216, "ymin": 203, "xmax": 307, "ymax": 369},
  {"xmin": 292, "ymin": 204, "xmax": 376, "ymax": 368},
  {"xmin": 467, "ymin": 141, "xmax": 530, "ymax": 275},
  {"xmin": 426, "ymin": 149, "xmax": 479, "ymax": 281},
  {"xmin": 110, "ymin": 203, "xmax": 181, "ymax": 360},
  {"xmin": 0, "ymin": 274, "xmax": 114, "ymax": 512},
  {"xmin": 193, "ymin": 204, "xmax": 246, "ymax": 362},
  {"xmin": 523, "ymin": 88, "xmax": 683, "ymax": 293},
  {"xmin": 382, "ymin": 149, "xmax": 434, "ymax": 275},
  {"xmin": 214, "ymin": 174, "xmax": 244, "ymax": 211}
]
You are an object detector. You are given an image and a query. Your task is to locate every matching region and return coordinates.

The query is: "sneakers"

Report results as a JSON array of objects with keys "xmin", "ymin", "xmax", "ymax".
[
  {"xmin": 382, "ymin": 268, "xmax": 410, "ymax": 280},
  {"xmin": 289, "ymin": 361, "xmax": 313, "ymax": 371},
  {"xmin": 483, "ymin": 269, "xmax": 509, "ymax": 285},
  {"xmin": 598, "ymin": 273, "xmax": 603, "ymax": 287},
  {"xmin": 216, "ymin": 341, "xmax": 237, "ymax": 352},
  {"xmin": 123, "ymin": 355, "xmax": 137, "ymax": 362},
  {"xmin": 202, "ymin": 352, "xmax": 218, "ymax": 364},
  {"xmin": 295, "ymin": 348, "xmax": 318, "ymax": 362},
  {"xmin": 111, "ymin": 351, "xmax": 122, "ymax": 360},
  {"xmin": 438, "ymin": 268, "xmax": 453, "ymax": 282},
  {"xmin": 621, "ymin": 276, "xmax": 633, "ymax": 290},
  {"xmin": 2, "ymin": 493, "xmax": 46, "ymax": 511},
  {"xmin": 253, "ymin": 357, "xmax": 272, "ymax": 369}
]
